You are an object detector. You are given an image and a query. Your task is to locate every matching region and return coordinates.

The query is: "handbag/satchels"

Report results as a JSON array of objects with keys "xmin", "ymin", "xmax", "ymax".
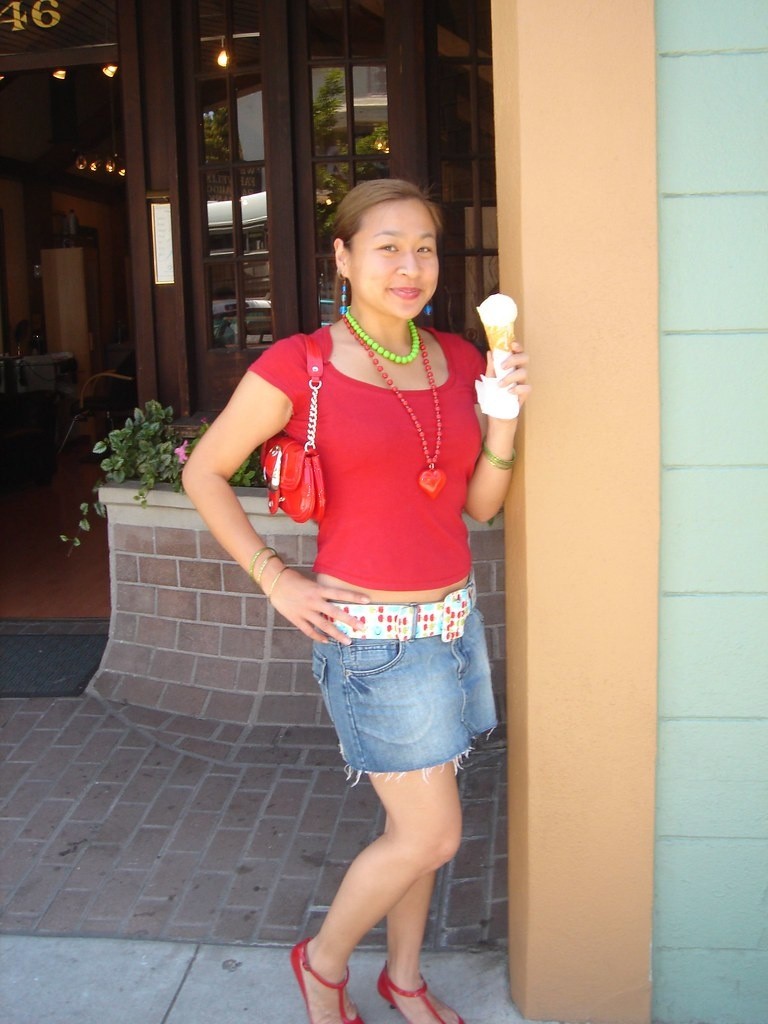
[{"xmin": 260, "ymin": 334, "xmax": 326, "ymax": 522}]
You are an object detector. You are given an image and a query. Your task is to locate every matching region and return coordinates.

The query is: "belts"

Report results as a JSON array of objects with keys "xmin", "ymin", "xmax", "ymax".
[{"xmin": 320, "ymin": 567, "xmax": 477, "ymax": 643}]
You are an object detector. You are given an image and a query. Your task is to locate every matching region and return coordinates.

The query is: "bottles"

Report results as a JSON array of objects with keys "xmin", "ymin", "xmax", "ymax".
[{"xmin": 66, "ymin": 208, "xmax": 79, "ymax": 233}]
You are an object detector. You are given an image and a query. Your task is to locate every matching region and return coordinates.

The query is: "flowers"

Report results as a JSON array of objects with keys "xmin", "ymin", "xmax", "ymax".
[{"xmin": 60, "ymin": 397, "xmax": 271, "ymax": 558}]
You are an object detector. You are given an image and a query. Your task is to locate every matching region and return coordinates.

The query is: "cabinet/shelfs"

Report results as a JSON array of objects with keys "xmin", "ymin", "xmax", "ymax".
[{"xmin": 41, "ymin": 242, "xmax": 139, "ymax": 402}]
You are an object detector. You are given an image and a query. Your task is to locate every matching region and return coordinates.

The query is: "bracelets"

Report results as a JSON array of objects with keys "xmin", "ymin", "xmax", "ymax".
[
  {"xmin": 249, "ymin": 547, "xmax": 289, "ymax": 605},
  {"xmin": 482, "ymin": 436, "xmax": 516, "ymax": 471}
]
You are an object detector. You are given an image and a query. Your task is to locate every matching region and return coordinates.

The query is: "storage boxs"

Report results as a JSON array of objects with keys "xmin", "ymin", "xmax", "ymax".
[{"xmin": 0, "ymin": 354, "xmax": 57, "ymax": 395}]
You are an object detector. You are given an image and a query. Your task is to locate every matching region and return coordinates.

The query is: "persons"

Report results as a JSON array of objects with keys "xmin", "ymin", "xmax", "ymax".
[{"xmin": 180, "ymin": 180, "xmax": 535, "ymax": 1024}]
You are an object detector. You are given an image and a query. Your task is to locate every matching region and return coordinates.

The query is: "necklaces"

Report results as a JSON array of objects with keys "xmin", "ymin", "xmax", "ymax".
[{"xmin": 342, "ymin": 305, "xmax": 448, "ymax": 501}]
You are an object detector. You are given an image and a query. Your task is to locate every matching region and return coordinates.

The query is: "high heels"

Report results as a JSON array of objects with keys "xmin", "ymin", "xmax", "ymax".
[
  {"xmin": 377, "ymin": 960, "xmax": 465, "ymax": 1024},
  {"xmin": 291, "ymin": 937, "xmax": 366, "ymax": 1024}
]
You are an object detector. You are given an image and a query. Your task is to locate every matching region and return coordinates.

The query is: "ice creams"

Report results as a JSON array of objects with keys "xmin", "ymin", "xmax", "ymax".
[{"xmin": 476, "ymin": 294, "xmax": 517, "ymax": 351}]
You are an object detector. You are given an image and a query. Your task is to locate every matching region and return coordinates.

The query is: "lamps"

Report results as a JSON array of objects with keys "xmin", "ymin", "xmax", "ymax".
[
  {"xmin": 49, "ymin": 67, "xmax": 67, "ymax": 80},
  {"xmin": 72, "ymin": 77, "xmax": 126, "ymax": 177},
  {"xmin": 94, "ymin": 62, "xmax": 120, "ymax": 78},
  {"xmin": 216, "ymin": 37, "xmax": 229, "ymax": 67}
]
